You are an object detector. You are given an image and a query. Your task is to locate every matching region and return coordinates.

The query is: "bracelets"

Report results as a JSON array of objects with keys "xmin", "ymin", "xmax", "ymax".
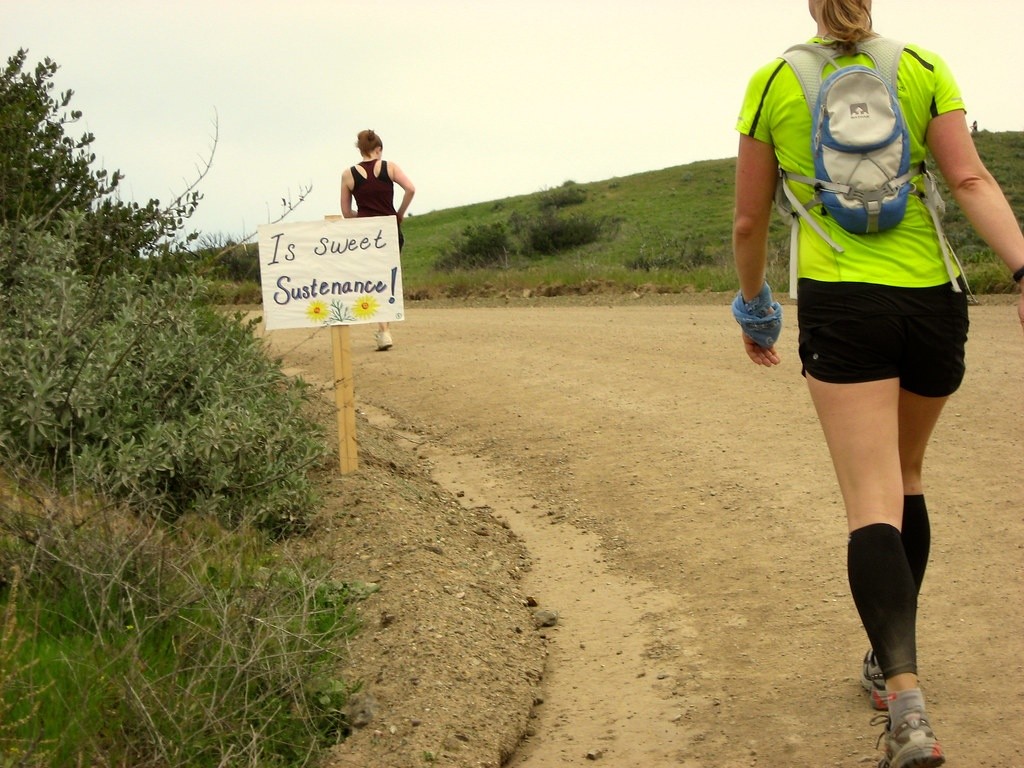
[{"xmin": 1013, "ymin": 267, "xmax": 1024, "ymax": 282}]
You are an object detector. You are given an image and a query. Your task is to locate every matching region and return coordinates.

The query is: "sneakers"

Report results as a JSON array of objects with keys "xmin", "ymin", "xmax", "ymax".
[
  {"xmin": 862, "ymin": 648, "xmax": 890, "ymax": 711},
  {"xmin": 869, "ymin": 715, "xmax": 945, "ymax": 768},
  {"xmin": 373, "ymin": 331, "xmax": 393, "ymax": 351}
]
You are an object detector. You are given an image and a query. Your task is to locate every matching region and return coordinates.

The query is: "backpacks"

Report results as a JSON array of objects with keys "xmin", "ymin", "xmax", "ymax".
[{"xmin": 774, "ymin": 37, "xmax": 930, "ymax": 235}]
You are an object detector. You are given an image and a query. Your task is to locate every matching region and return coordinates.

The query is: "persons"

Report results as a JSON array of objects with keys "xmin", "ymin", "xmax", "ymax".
[
  {"xmin": 340, "ymin": 130, "xmax": 416, "ymax": 351},
  {"xmin": 732, "ymin": 0, "xmax": 1024, "ymax": 768}
]
